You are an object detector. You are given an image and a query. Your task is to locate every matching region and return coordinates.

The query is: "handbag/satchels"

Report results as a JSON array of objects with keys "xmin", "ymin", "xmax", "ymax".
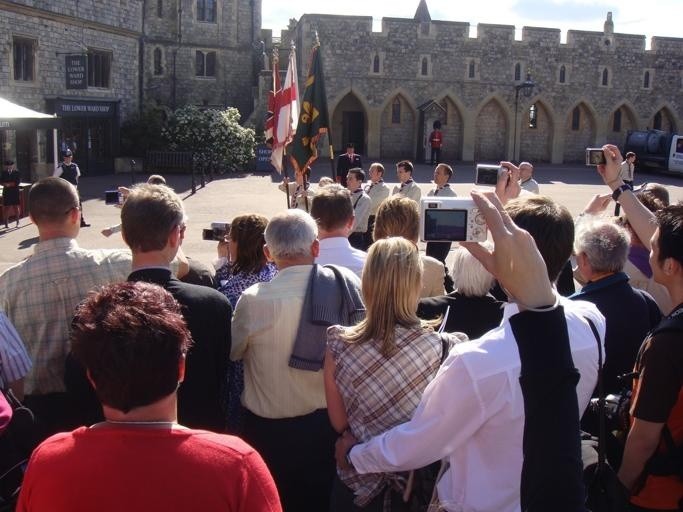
[
  {"xmin": 1, "ymin": 403, "xmax": 39, "ymax": 511},
  {"xmin": 581, "ymin": 437, "xmax": 631, "ymax": 512},
  {"xmin": 406, "ymin": 459, "xmax": 443, "ymax": 511}
]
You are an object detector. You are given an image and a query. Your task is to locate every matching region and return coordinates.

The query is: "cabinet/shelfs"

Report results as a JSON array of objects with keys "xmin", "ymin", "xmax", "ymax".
[
  {"xmin": 0, "ymin": 188, "xmax": 24, "ymax": 224},
  {"xmin": 18, "ymin": 183, "xmax": 32, "ymax": 217}
]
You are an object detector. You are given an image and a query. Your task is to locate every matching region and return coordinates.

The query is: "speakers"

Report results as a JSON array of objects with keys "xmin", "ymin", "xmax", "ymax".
[{"xmin": 105, "ymin": 191, "xmax": 119, "ymax": 203}]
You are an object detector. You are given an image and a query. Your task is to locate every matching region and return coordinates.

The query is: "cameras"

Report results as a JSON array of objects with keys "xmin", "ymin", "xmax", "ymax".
[
  {"xmin": 587, "ymin": 147, "xmax": 607, "ymax": 166},
  {"xmin": 203, "ymin": 223, "xmax": 228, "ymax": 240},
  {"xmin": 475, "ymin": 164, "xmax": 507, "ymax": 186},
  {"xmin": 420, "ymin": 197, "xmax": 488, "ymax": 241}
]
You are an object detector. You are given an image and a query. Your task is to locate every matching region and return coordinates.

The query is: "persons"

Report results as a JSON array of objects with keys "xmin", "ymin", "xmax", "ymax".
[{"xmin": 428, "ymin": 128, "xmax": 443, "ymax": 165}]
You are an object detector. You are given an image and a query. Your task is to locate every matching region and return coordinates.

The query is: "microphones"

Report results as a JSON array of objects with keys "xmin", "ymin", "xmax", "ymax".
[{"xmin": 131, "ymin": 159, "xmax": 136, "ymax": 167}]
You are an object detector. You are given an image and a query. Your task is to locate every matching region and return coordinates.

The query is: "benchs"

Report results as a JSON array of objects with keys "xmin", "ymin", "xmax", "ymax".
[{"xmin": 129, "ymin": 149, "xmax": 205, "ymax": 194}]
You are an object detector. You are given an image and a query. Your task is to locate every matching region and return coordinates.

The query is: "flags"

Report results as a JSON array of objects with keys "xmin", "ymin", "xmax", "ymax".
[
  {"xmin": 263, "ymin": 64, "xmax": 283, "ymax": 148},
  {"xmin": 270, "ymin": 54, "xmax": 300, "ymax": 175},
  {"xmin": 292, "ymin": 45, "xmax": 330, "ymax": 176}
]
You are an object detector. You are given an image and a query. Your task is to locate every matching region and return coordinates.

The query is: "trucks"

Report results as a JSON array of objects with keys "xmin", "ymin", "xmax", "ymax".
[{"xmin": 623, "ymin": 129, "xmax": 682, "ymax": 179}]
